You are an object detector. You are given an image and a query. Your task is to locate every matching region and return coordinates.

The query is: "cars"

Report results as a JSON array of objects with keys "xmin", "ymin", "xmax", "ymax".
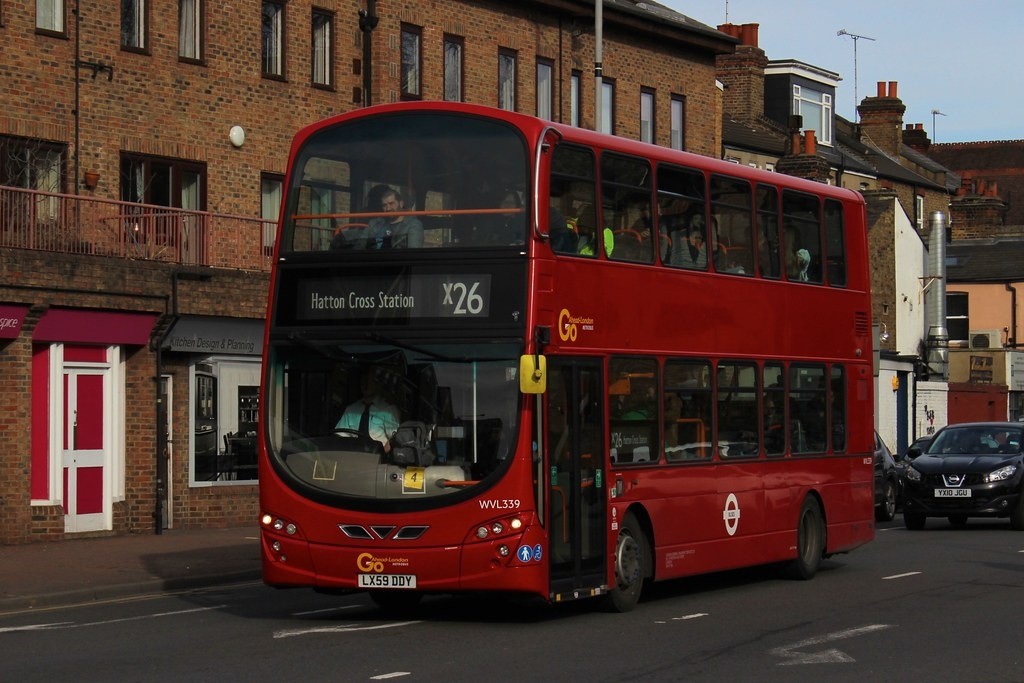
[
  {"xmin": 901, "ymin": 422, "xmax": 1024, "ymax": 530},
  {"xmin": 874, "ymin": 427, "xmax": 899, "ymax": 522}
]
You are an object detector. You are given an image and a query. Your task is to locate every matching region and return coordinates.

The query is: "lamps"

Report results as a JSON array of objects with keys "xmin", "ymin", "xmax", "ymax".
[
  {"xmin": 229, "ymin": 126, "xmax": 244, "ymax": 146},
  {"xmin": 879, "ymin": 321, "xmax": 889, "ymax": 344}
]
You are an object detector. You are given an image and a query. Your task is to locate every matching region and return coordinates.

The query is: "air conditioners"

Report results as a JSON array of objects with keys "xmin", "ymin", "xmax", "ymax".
[{"xmin": 969, "ymin": 329, "xmax": 1003, "ymax": 349}]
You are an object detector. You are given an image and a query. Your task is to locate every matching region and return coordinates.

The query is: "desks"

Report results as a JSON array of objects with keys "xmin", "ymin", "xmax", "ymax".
[{"xmin": 228, "ymin": 430, "xmax": 257, "ymax": 479}]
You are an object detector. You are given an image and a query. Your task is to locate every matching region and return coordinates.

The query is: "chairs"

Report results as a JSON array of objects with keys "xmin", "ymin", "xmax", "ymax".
[
  {"xmin": 216, "ymin": 421, "xmax": 259, "ymax": 480},
  {"xmin": 779, "ymin": 418, "xmax": 803, "ymax": 453},
  {"xmin": 614, "ymin": 229, "xmax": 754, "ymax": 276}
]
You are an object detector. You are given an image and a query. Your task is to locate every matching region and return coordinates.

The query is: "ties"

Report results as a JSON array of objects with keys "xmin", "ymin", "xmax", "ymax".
[{"xmin": 359, "ymin": 402, "xmax": 372, "ymax": 437}]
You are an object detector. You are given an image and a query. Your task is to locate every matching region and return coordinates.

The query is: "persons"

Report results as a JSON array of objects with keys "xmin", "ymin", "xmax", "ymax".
[
  {"xmin": 718, "ymin": 224, "xmax": 811, "ymax": 281},
  {"xmin": 482, "ymin": 191, "xmax": 526, "ymax": 241},
  {"xmin": 334, "ymin": 377, "xmax": 401, "ymax": 452},
  {"xmin": 490, "ymin": 384, "xmax": 518, "ymax": 439},
  {"xmin": 567, "ymin": 203, "xmax": 614, "ymax": 258},
  {"xmin": 803, "ymin": 389, "xmax": 843, "ymax": 450},
  {"xmin": 630, "ymin": 202, "xmax": 667, "ymax": 239},
  {"xmin": 621, "ymin": 382, "xmax": 682, "ymax": 462},
  {"xmin": 357, "ymin": 190, "xmax": 424, "ymax": 247},
  {"xmin": 509, "ymin": 192, "xmax": 569, "ymax": 250}
]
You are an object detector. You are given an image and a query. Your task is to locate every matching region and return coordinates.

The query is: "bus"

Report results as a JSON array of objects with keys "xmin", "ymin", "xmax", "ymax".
[{"xmin": 257, "ymin": 100, "xmax": 873, "ymax": 613}]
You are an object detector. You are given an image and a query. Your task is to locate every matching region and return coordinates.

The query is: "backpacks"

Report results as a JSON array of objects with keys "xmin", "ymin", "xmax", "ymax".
[{"xmin": 392, "ymin": 421, "xmax": 434, "ymax": 467}]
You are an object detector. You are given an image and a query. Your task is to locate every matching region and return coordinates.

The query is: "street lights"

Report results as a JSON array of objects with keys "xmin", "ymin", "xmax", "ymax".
[
  {"xmin": 838, "ymin": 29, "xmax": 875, "ymax": 139},
  {"xmin": 932, "ymin": 110, "xmax": 946, "ymax": 144}
]
